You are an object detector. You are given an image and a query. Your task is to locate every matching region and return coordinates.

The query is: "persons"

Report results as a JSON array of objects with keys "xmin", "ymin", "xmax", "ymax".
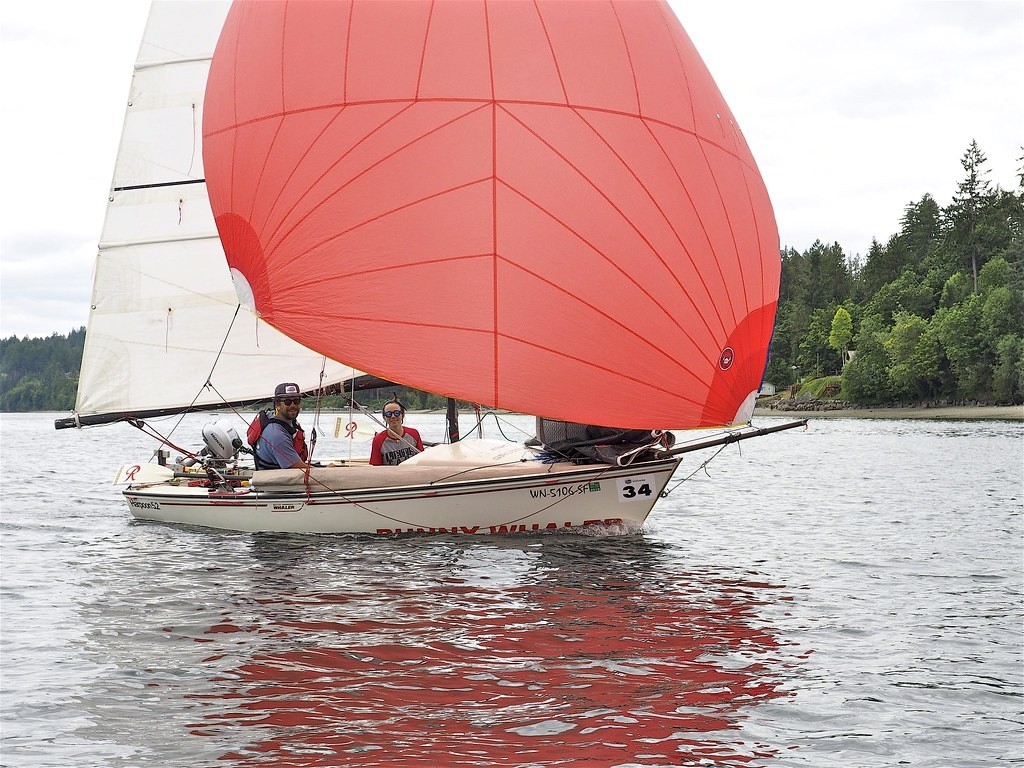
[
  {"xmin": 248, "ymin": 382, "xmax": 335, "ymax": 470},
  {"xmin": 369, "ymin": 400, "xmax": 425, "ymax": 468}
]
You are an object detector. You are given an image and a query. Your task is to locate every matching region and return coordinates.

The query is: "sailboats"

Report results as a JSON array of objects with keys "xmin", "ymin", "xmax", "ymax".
[{"xmin": 49, "ymin": 0, "xmax": 813, "ymax": 537}]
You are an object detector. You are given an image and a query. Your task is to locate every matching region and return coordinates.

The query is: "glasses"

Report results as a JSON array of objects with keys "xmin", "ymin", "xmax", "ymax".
[
  {"xmin": 383, "ymin": 410, "xmax": 402, "ymax": 418},
  {"xmin": 280, "ymin": 398, "xmax": 300, "ymax": 405}
]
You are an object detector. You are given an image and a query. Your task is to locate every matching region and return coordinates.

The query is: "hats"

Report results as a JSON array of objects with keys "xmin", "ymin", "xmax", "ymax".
[{"xmin": 273, "ymin": 383, "xmax": 303, "ymax": 402}]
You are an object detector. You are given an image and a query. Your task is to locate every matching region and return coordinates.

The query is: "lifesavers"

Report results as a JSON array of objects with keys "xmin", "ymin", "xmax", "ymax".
[{"xmin": 177, "ymin": 480, "xmax": 212, "ymax": 487}]
